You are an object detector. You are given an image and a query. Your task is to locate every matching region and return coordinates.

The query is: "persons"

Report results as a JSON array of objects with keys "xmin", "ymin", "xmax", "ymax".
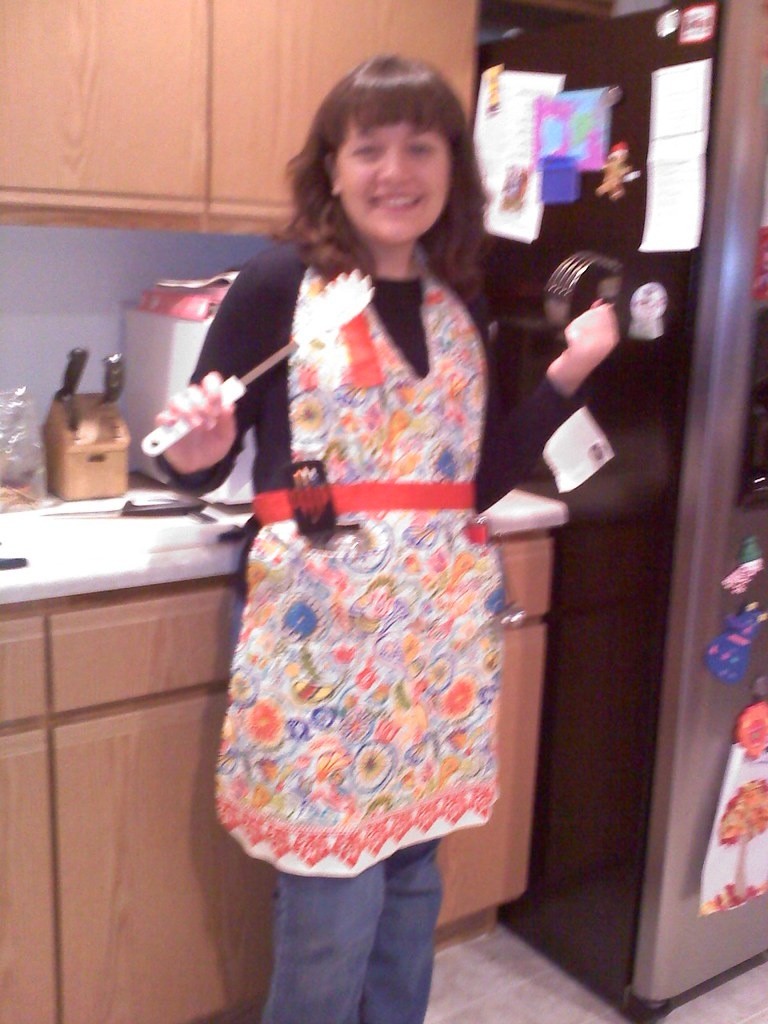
[{"xmin": 158, "ymin": 57, "xmax": 618, "ymax": 1024}]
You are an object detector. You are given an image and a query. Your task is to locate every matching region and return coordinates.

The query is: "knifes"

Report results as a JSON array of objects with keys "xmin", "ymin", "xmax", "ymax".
[
  {"xmin": 56, "ymin": 346, "xmax": 125, "ymax": 414},
  {"xmin": 40, "ymin": 499, "xmax": 208, "ymax": 517}
]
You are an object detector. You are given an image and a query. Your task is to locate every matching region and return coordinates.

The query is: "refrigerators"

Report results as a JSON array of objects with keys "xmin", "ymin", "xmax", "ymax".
[{"xmin": 463, "ymin": 0, "xmax": 768, "ymax": 1024}]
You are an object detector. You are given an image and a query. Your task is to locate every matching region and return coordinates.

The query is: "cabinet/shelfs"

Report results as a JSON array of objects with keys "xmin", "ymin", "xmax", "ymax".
[
  {"xmin": 0, "ymin": 0, "xmax": 481, "ymax": 231},
  {"xmin": 0, "ymin": 530, "xmax": 553, "ymax": 1024}
]
